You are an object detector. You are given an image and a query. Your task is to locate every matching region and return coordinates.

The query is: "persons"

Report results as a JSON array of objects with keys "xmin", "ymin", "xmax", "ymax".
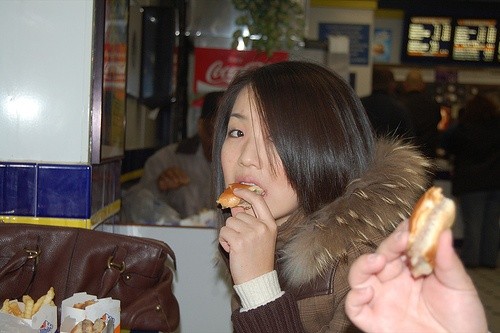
[
  {"xmin": 357, "ymin": 68, "xmax": 412, "ymax": 144},
  {"xmin": 210, "ymin": 59, "xmax": 427, "ymax": 333},
  {"xmin": 133, "ymin": 88, "xmax": 226, "ymax": 225},
  {"xmin": 447, "ymin": 90, "xmax": 500, "ymax": 268},
  {"xmin": 399, "ymin": 69, "xmax": 442, "ymax": 162},
  {"xmin": 345, "ymin": 187, "xmax": 491, "ymax": 333}
]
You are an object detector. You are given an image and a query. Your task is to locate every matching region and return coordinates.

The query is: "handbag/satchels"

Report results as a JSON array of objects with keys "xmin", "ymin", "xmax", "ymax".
[{"xmin": 0, "ymin": 222, "xmax": 180, "ymax": 333}]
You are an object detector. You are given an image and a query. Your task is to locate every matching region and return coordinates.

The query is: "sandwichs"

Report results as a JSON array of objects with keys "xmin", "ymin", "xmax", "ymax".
[
  {"xmin": 216, "ymin": 183, "xmax": 265, "ymax": 210},
  {"xmin": 404, "ymin": 186, "xmax": 455, "ymax": 277}
]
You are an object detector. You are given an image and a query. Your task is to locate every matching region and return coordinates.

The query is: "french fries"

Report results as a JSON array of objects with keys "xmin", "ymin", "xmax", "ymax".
[{"xmin": 1, "ymin": 286, "xmax": 55, "ymax": 319}]
